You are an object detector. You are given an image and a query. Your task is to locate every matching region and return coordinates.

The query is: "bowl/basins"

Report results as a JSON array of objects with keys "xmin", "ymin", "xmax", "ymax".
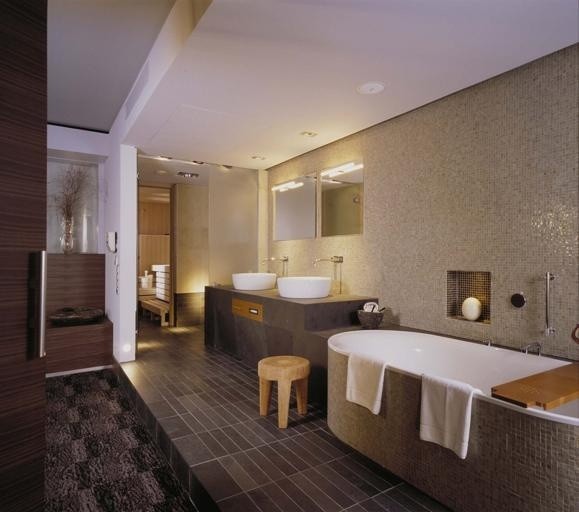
[{"xmin": 358, "ymin": 309, "xmax": 383, "ymax": 329}]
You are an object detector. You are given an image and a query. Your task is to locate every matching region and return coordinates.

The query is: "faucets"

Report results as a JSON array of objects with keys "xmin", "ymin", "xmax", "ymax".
[
  {"xmin": 311, "ymin": 256, "xmax": 344, "ymax": 264},
  {"xmin": 260, "ymin": 255, "xmax": 288, "ymax": 265},
  {"xmin": 519, "ymin": 342, "xmax": 542, "ymax": 357}
]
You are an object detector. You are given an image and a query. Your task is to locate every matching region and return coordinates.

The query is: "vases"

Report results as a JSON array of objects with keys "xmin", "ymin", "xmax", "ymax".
[{"xmin": 58, "ymin": 217, "xmax": 77, "ymax": 254}]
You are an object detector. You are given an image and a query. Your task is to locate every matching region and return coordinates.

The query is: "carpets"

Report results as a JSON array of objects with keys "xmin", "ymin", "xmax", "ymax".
[{"xmin": 39, "ymin": 364, "xmax": 199, "ymax": 511}]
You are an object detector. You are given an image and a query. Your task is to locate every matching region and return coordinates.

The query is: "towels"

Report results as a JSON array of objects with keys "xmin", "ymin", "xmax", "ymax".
[
  {"xmin": 418, "ymin": 371, "xmax": 485, "ymax": 462},
  {"xmin": 344, "ymin": 351, "xmax": 392, "ymax": 416}
]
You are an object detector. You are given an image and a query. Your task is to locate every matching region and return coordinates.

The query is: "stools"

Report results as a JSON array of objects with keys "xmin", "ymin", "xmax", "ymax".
[
  {"xmin": 141, "ymin": 297, "xmax": 172, "ymax": 327},
  {"xmin": 257, "ymin": 354, "xmax": 311, "ymax": 429}
]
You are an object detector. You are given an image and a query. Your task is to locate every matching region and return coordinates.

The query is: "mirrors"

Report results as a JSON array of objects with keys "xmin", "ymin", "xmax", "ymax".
[
  {"xmin": 321, "ymin": 156, "xmax": 365, "ymax": 238},
  {"xmin": 270, "ymin": 173, "xmax": 318, "ymax": 240}
]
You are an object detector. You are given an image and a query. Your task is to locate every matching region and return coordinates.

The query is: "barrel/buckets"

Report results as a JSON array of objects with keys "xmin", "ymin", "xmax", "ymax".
[{"xmin": 140, "ymin": 275, "xmax": 153, "ymax": 288}]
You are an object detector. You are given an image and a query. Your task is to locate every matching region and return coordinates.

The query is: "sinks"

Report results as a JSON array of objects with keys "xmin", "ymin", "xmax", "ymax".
[
  {"xmin": 231, "ymin": 272, "xmax": 277, "ymax": 291},
  {"xmin": 277, "ymin": 277, "xmax": 332, "ymax": 298}
]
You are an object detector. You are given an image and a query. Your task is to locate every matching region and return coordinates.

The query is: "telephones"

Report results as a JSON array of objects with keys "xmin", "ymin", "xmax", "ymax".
[{"xmin": 104, "ymin": 231, "xmax": 115, "ymax": 253}]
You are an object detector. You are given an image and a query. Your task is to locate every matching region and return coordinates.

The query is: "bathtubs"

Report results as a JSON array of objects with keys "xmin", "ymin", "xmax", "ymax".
[{"xmin": 326, "ymin": 328, "xmax": 578, "ymax": 512}]
